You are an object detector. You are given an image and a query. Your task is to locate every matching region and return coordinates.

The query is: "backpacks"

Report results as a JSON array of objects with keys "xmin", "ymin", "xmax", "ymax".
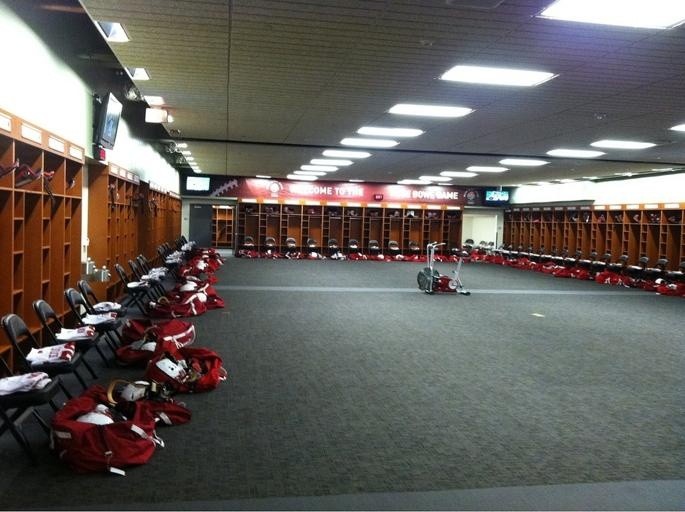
[
  {"xmin": 48, "ymin": 378, "xmax": 191, "ymax": 477},
  {"xmin": 237, "ymin": 248, "xmax": 503, "ymax": 265},
  {"xmin": 114, "ymin": 318, "xmax": 197, "ymax": 367},
  {"xmin": 503, "ymin": 256, "xmax": 685, "ymax": 298},
  {"xmin": 144, "ymin": 340, "xmax": 227, "ymax": 395},
  {"xmin": 147, "ymin": 247, "xmax": 226, "ymax": 316}
]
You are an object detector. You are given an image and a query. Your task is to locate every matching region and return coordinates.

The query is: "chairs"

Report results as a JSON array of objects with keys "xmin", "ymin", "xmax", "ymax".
[
  {"xmin": 140, "ymin": 254, "xmax": 167, "ymax": 292},
  {"xmin": 128, "ymin": 259, "xmax": 160, "ymax": 302},
  {"xmin": 114, "ymin": 263, "xmax": 155, "ymax": 316},
  {"xmin": 65, "ymin": 287, "xmax": 123, "ymax": 352},
  {"xmin": 464, "ymin": 239, "xmax": 685, "ymax": 286},
  {"xmin": 1, "ymin": 312, "xmax": 96, "ymax": 400},
  {"xmin": 158, "ymin": 236, "xmax": 199, "ymax": 281},
  {"xmin": 34, "ymin": 299, "xmax": 110, "ymax": 385},
  {"xmin": 0, "ymin": 357, "xmax": 73, "ymax": 455},
  {"xmin": 77, "ymin": 279, "xmax": 128, "ymax": 341},
  {"xmin": 136, "ymin": 256, "xmax": 165, "ymax": 297}
]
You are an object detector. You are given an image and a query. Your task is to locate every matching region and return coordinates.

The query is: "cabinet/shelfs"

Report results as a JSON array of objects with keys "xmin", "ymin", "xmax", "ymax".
[
  {"xmin": 210, "ymin": 202, "xmax": 464, "ymax": 258},
  {"xmin": 502, "ymin": 202, "xmax": 685, "ymax": 268}
]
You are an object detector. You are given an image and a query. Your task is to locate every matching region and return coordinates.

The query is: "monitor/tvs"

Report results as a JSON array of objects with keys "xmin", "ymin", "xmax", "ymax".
[
  {"xmin": 186, "ymin": 176, "xmax": 211, "ymax": 192},
  {"xmin": 93, "ymin": 93, "xmax": 124, "ymax": 150},
  {"xmin": 486, "ymin": 191, "xmax": 509, "ymax": 203}
]
